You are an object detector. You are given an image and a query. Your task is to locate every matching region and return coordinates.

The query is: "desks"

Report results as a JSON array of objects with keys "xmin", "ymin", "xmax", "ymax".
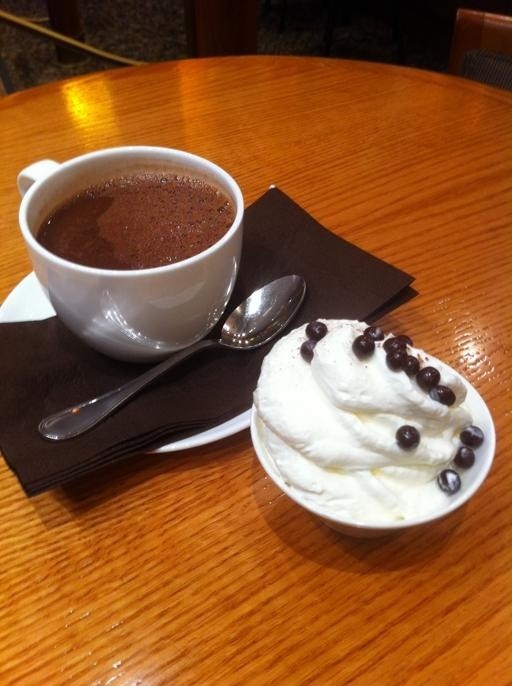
[{"xmin": 0, "ymin": 53, "xmax": 512, "ymax": 686}]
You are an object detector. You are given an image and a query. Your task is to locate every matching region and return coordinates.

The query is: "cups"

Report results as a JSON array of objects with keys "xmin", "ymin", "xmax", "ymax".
[{"xmin": 16, "ymin": 143, "xmax": 243, "ymax": 361}]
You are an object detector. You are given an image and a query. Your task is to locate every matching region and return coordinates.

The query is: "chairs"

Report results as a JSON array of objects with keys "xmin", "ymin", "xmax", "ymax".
[{"xmin": 446, "ymin": 8, "xmax": 512, "ymax": 76}]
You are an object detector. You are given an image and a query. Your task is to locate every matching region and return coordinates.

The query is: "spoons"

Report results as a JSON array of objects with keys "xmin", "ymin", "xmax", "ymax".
[{"xmin": 39, "ymin": 270, "xmax": 310, "ymax": 440}]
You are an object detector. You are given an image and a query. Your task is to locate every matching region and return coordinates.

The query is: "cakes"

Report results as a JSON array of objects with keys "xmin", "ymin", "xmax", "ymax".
[{"xmin": 251, "ymin": 317, "xmax": 495, "ymax": 538}]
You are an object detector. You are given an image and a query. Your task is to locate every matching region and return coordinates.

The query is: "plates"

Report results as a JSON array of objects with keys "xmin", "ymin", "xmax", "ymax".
[{"xmin": 0, "ymin": 265, "xmax": 253, "ymax": 459}]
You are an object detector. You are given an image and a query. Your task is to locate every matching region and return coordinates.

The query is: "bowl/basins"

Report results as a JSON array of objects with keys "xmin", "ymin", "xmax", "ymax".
[{"xmin": 253, "ymin": 348, "xmax": 500, "ymax": 535}]
